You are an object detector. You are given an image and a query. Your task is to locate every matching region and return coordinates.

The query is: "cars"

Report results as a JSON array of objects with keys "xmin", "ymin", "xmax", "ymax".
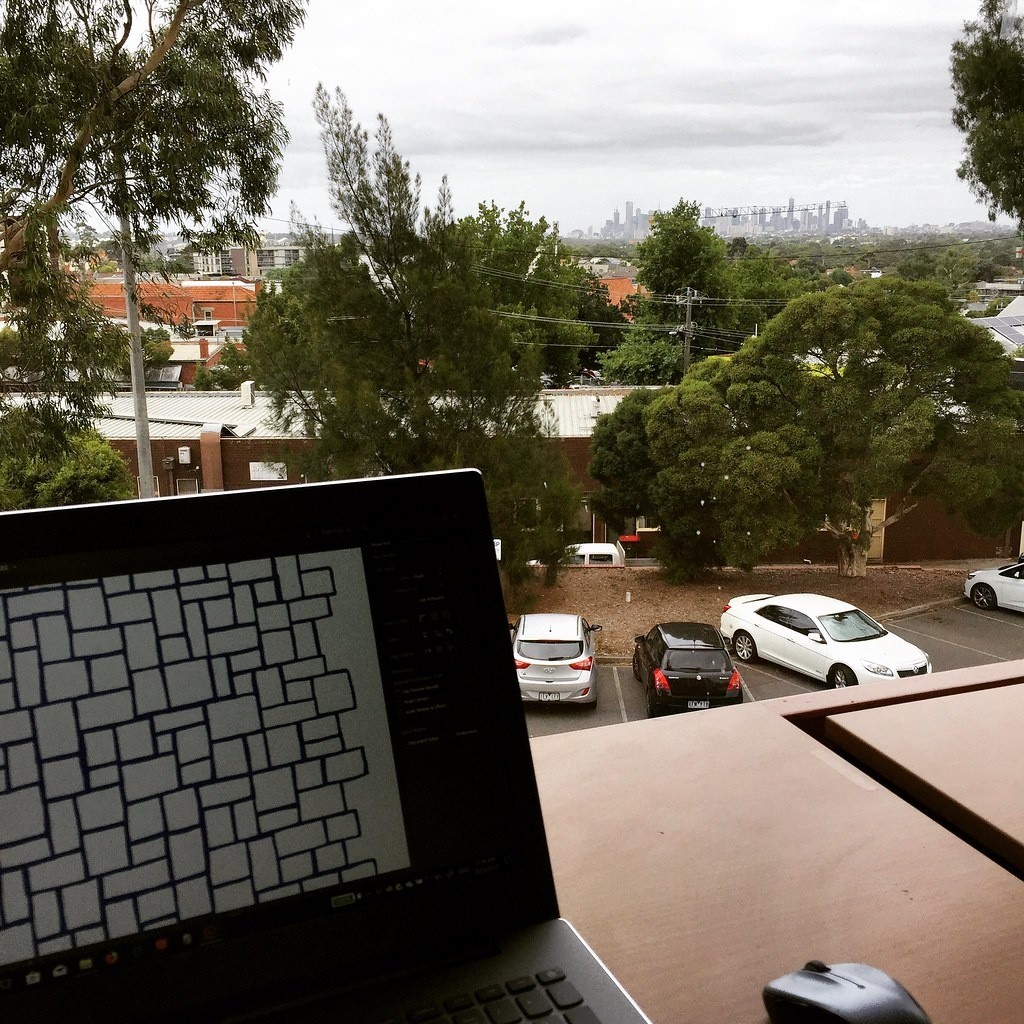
[
  {"xmin": 963, "ymin": 563, "xmax": 1024, "ymax": 614},
  {"xmin": 720, "ymin": 593, "xmax": 933, "ymax": 690},
  {"xmin": 508, "ymin": 613, "xmax": 603, "ymax": 710}
]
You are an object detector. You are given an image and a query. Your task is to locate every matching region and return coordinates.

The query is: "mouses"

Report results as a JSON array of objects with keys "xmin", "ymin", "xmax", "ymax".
[{"xmin": 763, "ymin": 960, "xmax": 933, "ymax": 1024}]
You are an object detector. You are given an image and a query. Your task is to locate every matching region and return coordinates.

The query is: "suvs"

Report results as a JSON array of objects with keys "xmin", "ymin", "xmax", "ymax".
[{"xmin": 632, "ymin": 622, "xmax": 743, "ymax": 719}]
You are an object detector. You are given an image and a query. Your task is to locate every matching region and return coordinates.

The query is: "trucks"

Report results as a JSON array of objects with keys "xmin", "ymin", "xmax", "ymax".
[{"xmin": 526, "ymin": 541, "xmax": 663, "ymax": 566}]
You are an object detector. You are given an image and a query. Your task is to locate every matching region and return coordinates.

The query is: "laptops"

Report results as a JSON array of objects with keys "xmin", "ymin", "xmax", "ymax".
[{"xmin": 0, "ymin": 467, "xmax": 652, "ymax": 1024}]
